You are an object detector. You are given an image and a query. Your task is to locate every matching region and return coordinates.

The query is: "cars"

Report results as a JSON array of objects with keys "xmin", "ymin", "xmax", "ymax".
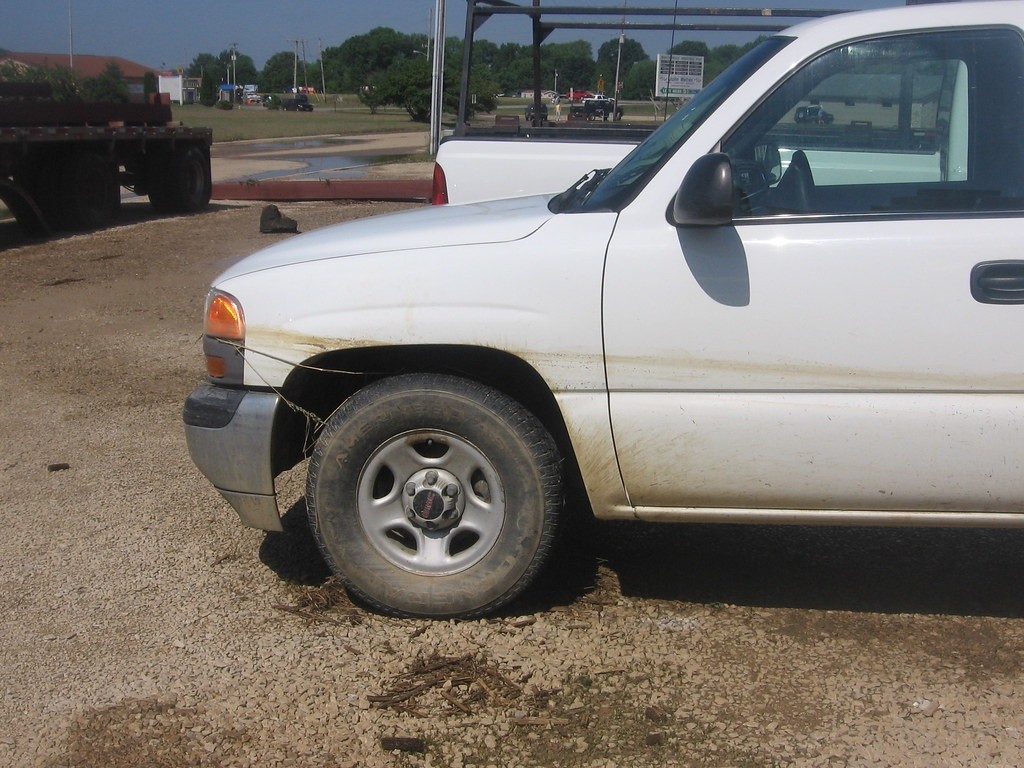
[
  {"xmin": 182, "ymin": 1, "xmax": 1023, "ymax": 622},
  {"xmin": 243, "ymin": 89, "xmax": 261, "ymax": 104}
]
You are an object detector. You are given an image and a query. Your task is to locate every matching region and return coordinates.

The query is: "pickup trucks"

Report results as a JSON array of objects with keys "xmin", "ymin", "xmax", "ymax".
[
  {"xmin": 524, "ymin": 101, "xmax": 548, "ymax": 121},
  {"xmin": 580, "ymin": 94, "xmax": 617, "ymax": 107},
  {"xmin": 570, "ymin": 99, "xmax": 624, "ymax": 121},
  {"xmin": 566, "ymin": 90, "xmax": 595, "ymax": 102},
  {"xmin": 280, "ymin": 93, "xmax": 313, "ymax": 112},
  {"xmin": 427, "ymin": 1, "xmax": 968, "ymax": 214}
]
